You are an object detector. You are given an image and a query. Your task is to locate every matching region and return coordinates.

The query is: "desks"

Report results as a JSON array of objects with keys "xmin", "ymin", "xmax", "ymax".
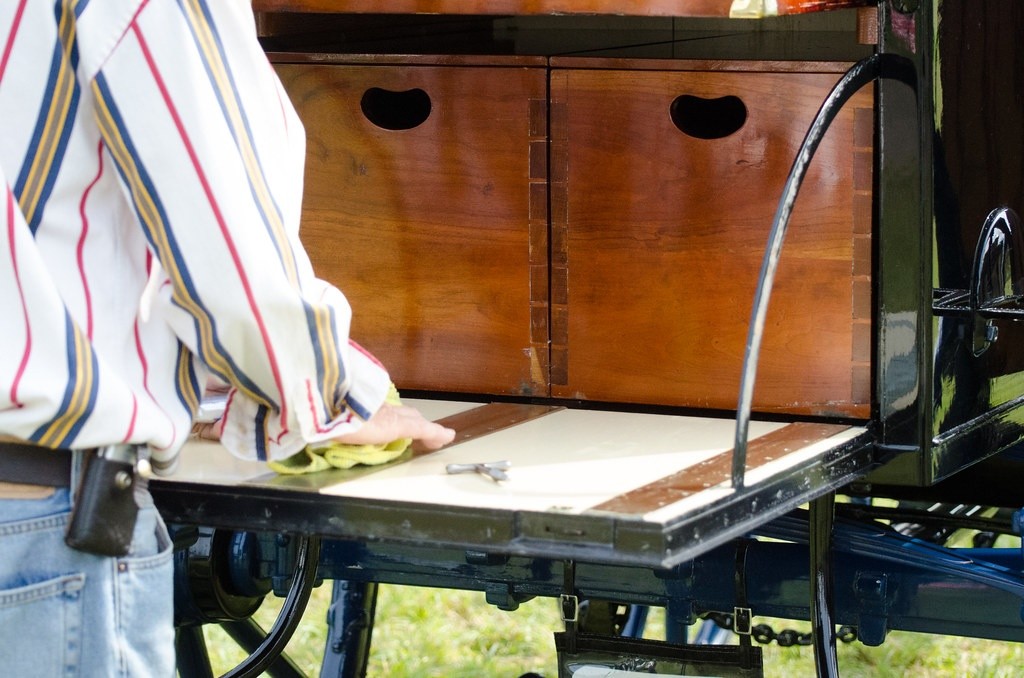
[{"xmin": 122, "ymin": 393, "xmax": 876, "ymax": 678}]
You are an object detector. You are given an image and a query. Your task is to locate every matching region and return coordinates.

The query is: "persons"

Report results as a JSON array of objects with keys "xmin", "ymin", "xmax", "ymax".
[{"xmin": 0, "ymin": 0, "xmax": 457, "ymax": 678}]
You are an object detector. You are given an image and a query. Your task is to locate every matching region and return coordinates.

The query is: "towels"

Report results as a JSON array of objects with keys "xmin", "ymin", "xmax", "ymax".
[{"xmin": 264, "ymin": 383, "xmax": 413, "ymax": 474}]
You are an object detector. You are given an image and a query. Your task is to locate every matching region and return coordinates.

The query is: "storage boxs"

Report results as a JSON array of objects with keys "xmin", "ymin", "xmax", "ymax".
[
  {"xmin": 549, "ymin": 53, "xmax": 876, "ymax": 421},
  {"xmin": 263, "ymin": 50, "xmax": 547, "ymax": 402}
]
